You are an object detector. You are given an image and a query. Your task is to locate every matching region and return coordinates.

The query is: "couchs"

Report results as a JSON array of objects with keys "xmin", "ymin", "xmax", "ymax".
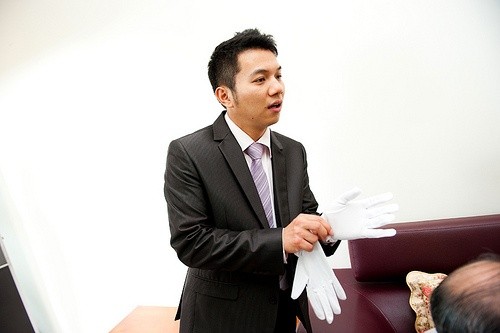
[{"xmin": 296, "ymin": 212, "xmax": 500, "ymax": 333}]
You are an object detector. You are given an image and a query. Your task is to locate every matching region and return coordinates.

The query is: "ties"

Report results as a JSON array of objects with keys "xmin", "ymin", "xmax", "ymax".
[{"xmin": 246, "ymin": 143, "xmax": 275, "ymax": 229}]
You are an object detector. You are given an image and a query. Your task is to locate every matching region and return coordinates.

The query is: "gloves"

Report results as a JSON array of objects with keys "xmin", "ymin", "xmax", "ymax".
[
  {"xmin": 320, "ymin": 188, "xmax": 397, "ymax": 243},
  {"xmin": 290, "ymin": 240, "xmax": 346, "ymax": 323}
]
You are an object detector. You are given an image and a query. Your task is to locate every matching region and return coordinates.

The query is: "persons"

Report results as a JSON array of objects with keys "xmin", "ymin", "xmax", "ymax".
[
  {"xmin": 430, "ymin": 253, "xmax": 500, "ymax": 332},
  {"xmin": 164, "ymin": 27, "xmax": 397, "ymax": 332}
]
